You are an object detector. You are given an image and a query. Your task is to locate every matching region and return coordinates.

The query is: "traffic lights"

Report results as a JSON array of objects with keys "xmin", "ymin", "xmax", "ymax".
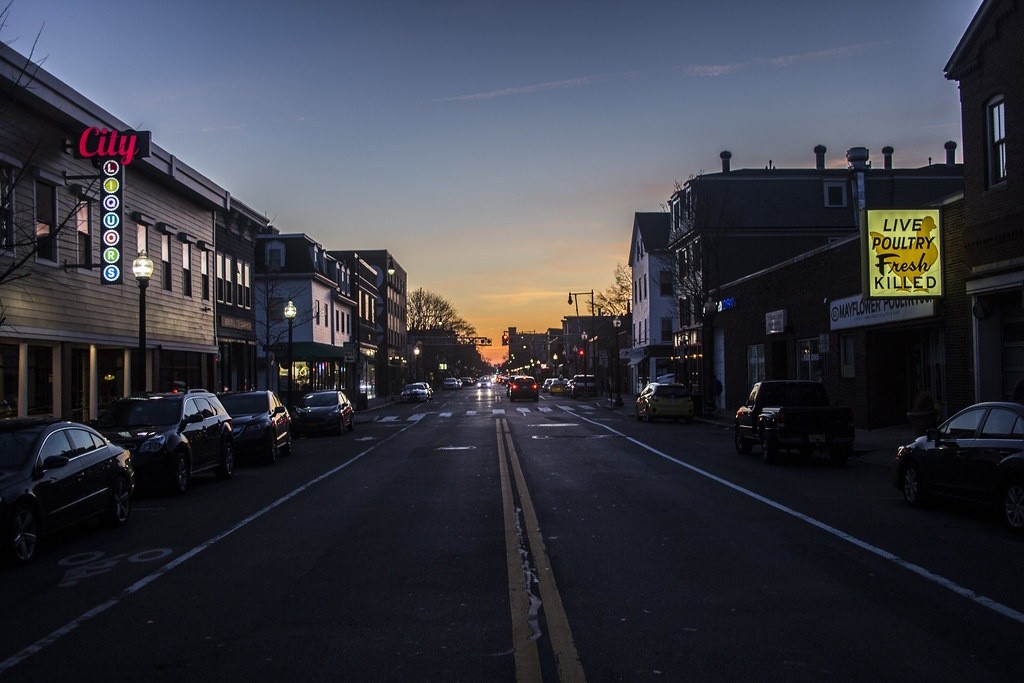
[
  {"xmin": 579, "ymin": 350, "xmax": 584, "ymax": 355},
  {"xmin": 503, "ymin": 335, "xmax": 508, "ymax": 345}
]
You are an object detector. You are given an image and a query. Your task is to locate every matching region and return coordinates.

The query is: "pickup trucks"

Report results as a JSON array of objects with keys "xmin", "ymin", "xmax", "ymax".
[{"xmin": 734, "ymin": 380, "xmax": 855, "ymax": 467}]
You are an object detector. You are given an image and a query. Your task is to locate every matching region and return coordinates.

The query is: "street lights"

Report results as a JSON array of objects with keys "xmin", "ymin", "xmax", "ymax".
[
  {"xmin": 284, "ymin": 298, "xmax": 297, "ymax": 417},
  {"xmin": 132, "ymin": 250, "xmax": 154, "ymax": 391},
  {"xmin": 580, "ymin": 330, "xmax": 588, "ymax": 394},
  {"xmin": 413, "ymin": 346, "xmax": 420, "ymax": 383},
  {"xmin": 553, "ymin": 353, "xmax": 558, "ymax": 378},
  {"xmin": 613, "ymin": 316, "xmax": 625, "ymax": 407},
  {"xmin": 568, "ymin": 289, "xmax": 598, "ymax": 397}
]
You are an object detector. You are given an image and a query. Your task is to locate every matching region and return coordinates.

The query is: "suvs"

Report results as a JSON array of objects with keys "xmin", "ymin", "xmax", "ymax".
[{"xmin": 85, "ymin": 389, "xmax": 236, "ymax": 498}]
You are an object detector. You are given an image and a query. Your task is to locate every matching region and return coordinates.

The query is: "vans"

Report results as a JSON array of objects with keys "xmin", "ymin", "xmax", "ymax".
[{"xmin": 570, "ymin": 374, "xmax": 598, "ymax": 399}]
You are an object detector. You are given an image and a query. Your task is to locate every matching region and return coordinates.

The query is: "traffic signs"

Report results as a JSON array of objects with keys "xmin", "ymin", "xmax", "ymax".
[{"xmin": 344, "ymin": 347, "xmax": 355, "ymax": 363}]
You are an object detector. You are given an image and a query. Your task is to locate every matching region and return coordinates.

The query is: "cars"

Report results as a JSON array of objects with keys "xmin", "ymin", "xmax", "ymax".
[
  {"xmin": 290, "ymin": 390, "xmax": 354, "ymax": 441},
  {"xmin": 0, "ymin": 417, "xmax": 136, "ymax": 570},
  {"xmin": 496, "ymin": 375, "xmax": 514, "ymax": 397},
  {"xmin": 508, "ymin": 376, "xmax": 539, "ymax": 402},
  {"xmin": 890, "ymin": 402, "xmax": 1024, "ymax": 542},
  {"xmin": 635, "ymin": 374, "xmax": 694, "ymax": 424},
  {"xmin": 400, "ymin": 381, "xmax": 433, "ymax": 403},
  {"xmin": 219, "ymin": 390, "xmax": 293, "ymax": 465},
  {"xmin": 443, "ymin": 376, "xmax": 473, "ymax": 390},
  {"xmin": 543, "ymin": 373, "xmax": 569, "ymax": 396}
]
